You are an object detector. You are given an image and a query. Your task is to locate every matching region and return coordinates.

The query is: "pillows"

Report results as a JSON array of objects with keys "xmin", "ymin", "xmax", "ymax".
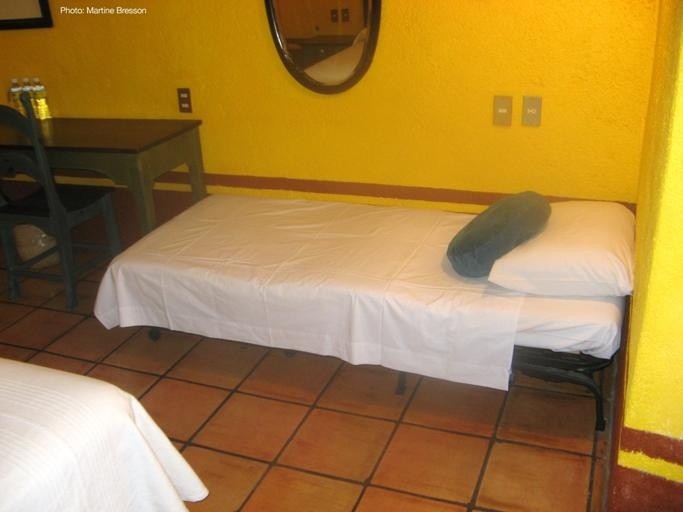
[
  {"xmin": 445, "ymin": 189, "xmax": 552, "ymax": 278},
  {"xmin": 486, "ymin": 199, "xmax": 636, "ymax": 299}
]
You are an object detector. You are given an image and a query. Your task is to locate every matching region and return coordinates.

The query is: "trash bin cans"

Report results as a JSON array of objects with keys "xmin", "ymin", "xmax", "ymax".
[{"xmin": 14, "ymin": 223, "xmax": 60, "ymax": 269}]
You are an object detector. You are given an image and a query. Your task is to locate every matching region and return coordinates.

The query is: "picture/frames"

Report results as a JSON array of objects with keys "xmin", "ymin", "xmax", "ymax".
[{"xmin": 0, "ymin": 0, "xmax": 54, "ymax": 30}]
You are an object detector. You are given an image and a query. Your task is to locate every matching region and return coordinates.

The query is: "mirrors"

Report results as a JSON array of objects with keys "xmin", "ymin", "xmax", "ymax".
[{"xmin": 265, "ymin": 0, "xmax": 382, "ymax": 96}]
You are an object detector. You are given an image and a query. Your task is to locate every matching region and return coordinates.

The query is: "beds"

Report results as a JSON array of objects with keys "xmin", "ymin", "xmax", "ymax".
[
  {"xmin": 303, "ymin": 46, "xmax": 364, "ymax": 86},
  {"xmin": 93, "ymin": 191, "xmax": 628, "ymax": 431},
  {"xmin": 0, "ymin": 356, "xmax": 213, "ymax": 512}
]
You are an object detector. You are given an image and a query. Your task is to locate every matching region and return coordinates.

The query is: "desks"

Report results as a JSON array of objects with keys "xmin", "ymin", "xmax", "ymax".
[
  {"xmin": 0, "ymin": 116, "xmax": 211, "ymax": 342},
  {"xmin": 304, "ymin": 34, "xmax": 354, "ymax": 66}
]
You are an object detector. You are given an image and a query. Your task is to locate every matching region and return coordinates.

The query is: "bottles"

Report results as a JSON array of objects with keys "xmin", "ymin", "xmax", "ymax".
[{"xmin": 9, "ymin": 77, "xmax": 51, "ymax": 121}]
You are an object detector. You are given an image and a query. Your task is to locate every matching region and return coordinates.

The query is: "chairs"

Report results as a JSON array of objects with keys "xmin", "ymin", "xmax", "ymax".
[{"xmin": 0, "ymin": 90, "xmax": 124, "ymax": 310}]
